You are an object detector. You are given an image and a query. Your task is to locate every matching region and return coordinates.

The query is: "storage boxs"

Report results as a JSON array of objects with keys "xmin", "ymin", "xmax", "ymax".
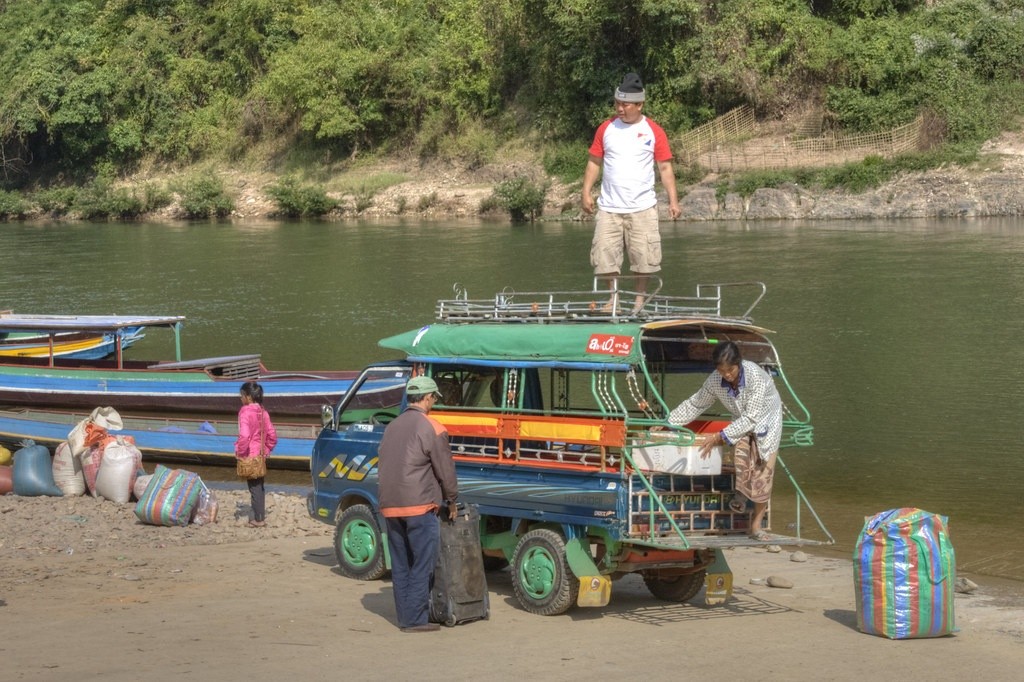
[{"xmin": 631, "ymin": 430, "xmax": 722, "ymax": 477}]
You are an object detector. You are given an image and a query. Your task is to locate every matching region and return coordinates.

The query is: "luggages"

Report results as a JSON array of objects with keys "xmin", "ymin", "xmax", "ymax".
[{"xmin": 428, "ymin": 502, "xmax": 491, "ymax": 627}]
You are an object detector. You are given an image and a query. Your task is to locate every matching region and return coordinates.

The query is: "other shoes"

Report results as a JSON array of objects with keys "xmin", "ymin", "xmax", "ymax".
[{"xmin": 401, "ymin": 623, "xmax": 441, "ymax": 633}]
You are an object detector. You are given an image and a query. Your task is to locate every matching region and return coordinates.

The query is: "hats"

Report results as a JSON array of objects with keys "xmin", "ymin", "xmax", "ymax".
[
  {"xmin": 614, "ymin": 73, "xmax": 646, "ymax": 103},
  {"xmin": 406, "ymin": 376, "xmax": 442, "ymax": 397}
]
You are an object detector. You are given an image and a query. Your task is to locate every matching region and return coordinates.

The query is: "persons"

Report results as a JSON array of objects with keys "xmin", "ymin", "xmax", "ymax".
[
  {"xmin": 234, "ymin": 381, "xmax": 277, "ymax": 528},
  {"xmin": 376, "ymin": 376, "xmax": 458, "ymax": 632},
  {"xmin": 649, "ymin": 342, "xmax": 782, "ymax": 542},
  {"xmin": 581, "ymin": 73, "xmax": 681, "ymax": 314}
]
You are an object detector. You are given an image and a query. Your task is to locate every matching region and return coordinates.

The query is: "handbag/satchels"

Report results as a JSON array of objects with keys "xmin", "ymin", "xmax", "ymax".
[
  {"xmin": 436, "ymin": 372, "xmax": 462, "ymax": 406},
  {"xmin": 133, "ymin": 464, "xmax": 206, "ymax": 527},
  {"xmin": 237, "ymin": 454, "xmax": 266, "ymax": 479},
  {"xmin": 853, "ymin": 507, "xmax": 962, "ymax": 640}
]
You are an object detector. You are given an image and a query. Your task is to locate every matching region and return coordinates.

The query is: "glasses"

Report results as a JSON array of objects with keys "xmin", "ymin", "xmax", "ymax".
[{"xmin": 433, "ymin": 395, "xmax": 438, "ymax": 402}]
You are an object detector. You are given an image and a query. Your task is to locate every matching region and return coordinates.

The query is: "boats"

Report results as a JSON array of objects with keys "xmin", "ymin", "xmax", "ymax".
[{"xmin": 0, "ymin": 313, "xmax": 498, "ymax": 470}]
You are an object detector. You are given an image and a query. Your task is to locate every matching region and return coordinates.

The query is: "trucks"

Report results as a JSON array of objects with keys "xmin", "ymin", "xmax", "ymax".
[{"xmin": 305, "ymin": 361, "xmax": 765, "ymax": 615}]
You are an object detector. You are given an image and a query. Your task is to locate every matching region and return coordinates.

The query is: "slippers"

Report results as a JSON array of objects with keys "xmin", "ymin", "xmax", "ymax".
[
  {"xmin": 245, "ymin": 520, "xmax": 268, "ymax": 528},
  {"xmin": 729, "ymin": 491, "xmax": 747, "ymax": 513},
  {"xmin": 749, "ymin": 531, "xmax": 771, "ymax": 541}
]
